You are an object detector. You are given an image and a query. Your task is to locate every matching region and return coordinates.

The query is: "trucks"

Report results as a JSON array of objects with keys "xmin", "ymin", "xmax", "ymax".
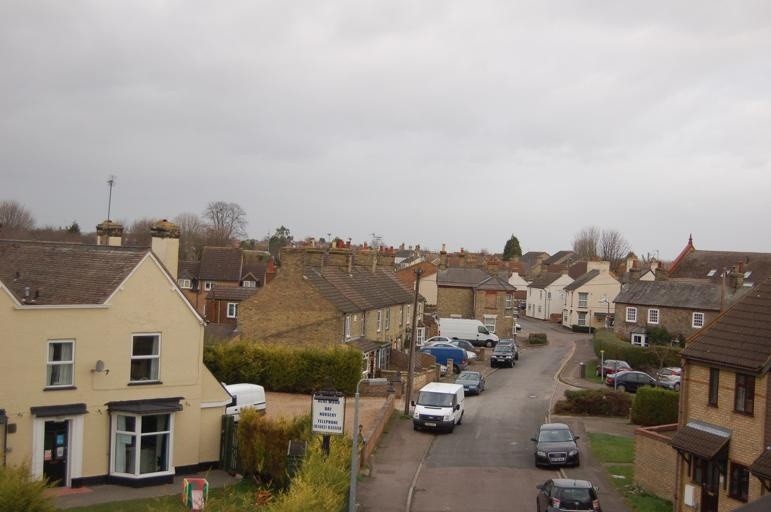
[
  {"xmin": 519, "ymin": 300, "xmax": 526, "ymax": 312},
  {"xmin": 513, "ymin": 306, "xmax": 520, "ymax": 317},
  {"xmin": 605, "ymin": 314, "xmax": 616, "ymax": 329}
]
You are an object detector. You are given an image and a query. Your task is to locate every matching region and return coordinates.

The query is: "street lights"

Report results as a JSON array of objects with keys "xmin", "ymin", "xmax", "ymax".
[
  {"xmin": 599, "ymin": 349, "xmax": 605, "ymax": 384},
  {"xmin": 349, "ymin": 377, "xmax": 389, "ymax": 512}
]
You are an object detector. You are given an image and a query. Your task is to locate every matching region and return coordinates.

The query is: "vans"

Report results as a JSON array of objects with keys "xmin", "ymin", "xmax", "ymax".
[
  {"xmin": 411, "ymin": 380, "xmax": 468, "ymax": 433},
  {"xmin": 218, "ymin": 378, "xmax": 269, "ymax": 427}
]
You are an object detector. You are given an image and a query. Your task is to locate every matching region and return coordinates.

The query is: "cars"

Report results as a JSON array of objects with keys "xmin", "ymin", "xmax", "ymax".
[
  {"xmin": 455, "ymin": 370, "xmax": 487, "ymax": 394},
  {"xmin": 531, "ymin": 422, "xmax": 580, "ymax": 470},
  {"xmin": 420, "ymin": 315, "xmax": 519, "ymax": 372},
  {"xmin": 596, "ymin": 359, "xmax": 681, "ymax": 393},
  {"xmin": 516, "ymin": 324, "xmax": 521, "ymax": 331}
]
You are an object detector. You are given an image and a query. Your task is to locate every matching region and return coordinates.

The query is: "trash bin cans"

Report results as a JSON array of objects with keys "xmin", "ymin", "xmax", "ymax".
[{"xmin": 580, "ymin": 363, "xmax": 585, "ymax": 378}]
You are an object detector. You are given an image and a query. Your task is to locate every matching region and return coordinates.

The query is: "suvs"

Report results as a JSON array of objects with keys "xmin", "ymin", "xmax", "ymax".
[{"xmin": 535, "ymin": 477, "xmax": 604, "ymax": 511}]
